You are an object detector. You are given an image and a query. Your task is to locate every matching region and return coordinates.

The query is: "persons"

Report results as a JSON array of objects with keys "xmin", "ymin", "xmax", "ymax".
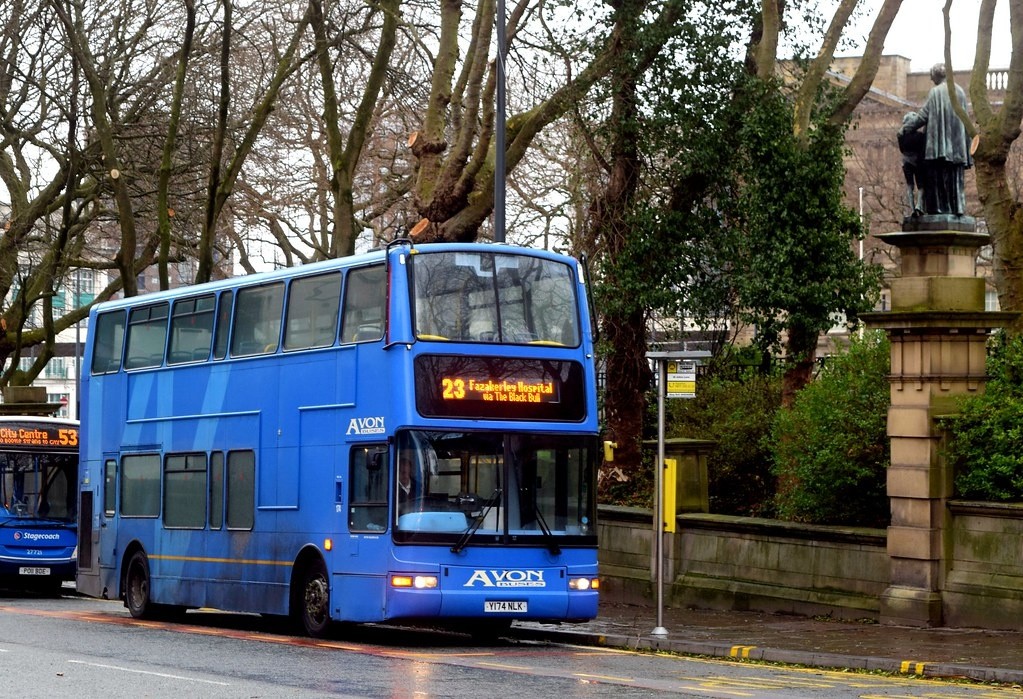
[
  {"xmin": 897, "ymin": 62, "xmax": 972, "ymax": 217},
  {"xmin": 398, "ymin": 458, "xmax": 421, "ymax": 516}
]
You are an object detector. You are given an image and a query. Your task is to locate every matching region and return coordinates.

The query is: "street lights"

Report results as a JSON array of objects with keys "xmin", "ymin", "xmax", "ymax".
[{"xmin": 646, "ymin": 351, "xmax": 714, "ymax": 633}]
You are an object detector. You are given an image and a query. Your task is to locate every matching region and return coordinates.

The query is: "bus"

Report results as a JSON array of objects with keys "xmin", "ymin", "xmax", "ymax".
[
  {"xmin": 1, "ymin": 415, "xmax": 81, "ymax": 597},
  {"xmin": 75, "ymin": 238, "xmax": 618, "ymax": 635}
]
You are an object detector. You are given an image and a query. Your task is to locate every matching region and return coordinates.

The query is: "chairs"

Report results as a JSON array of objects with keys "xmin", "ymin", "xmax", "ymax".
[{"xmin": 106, "ymin": 323, "xmax": 380, "ymax": 373}]
